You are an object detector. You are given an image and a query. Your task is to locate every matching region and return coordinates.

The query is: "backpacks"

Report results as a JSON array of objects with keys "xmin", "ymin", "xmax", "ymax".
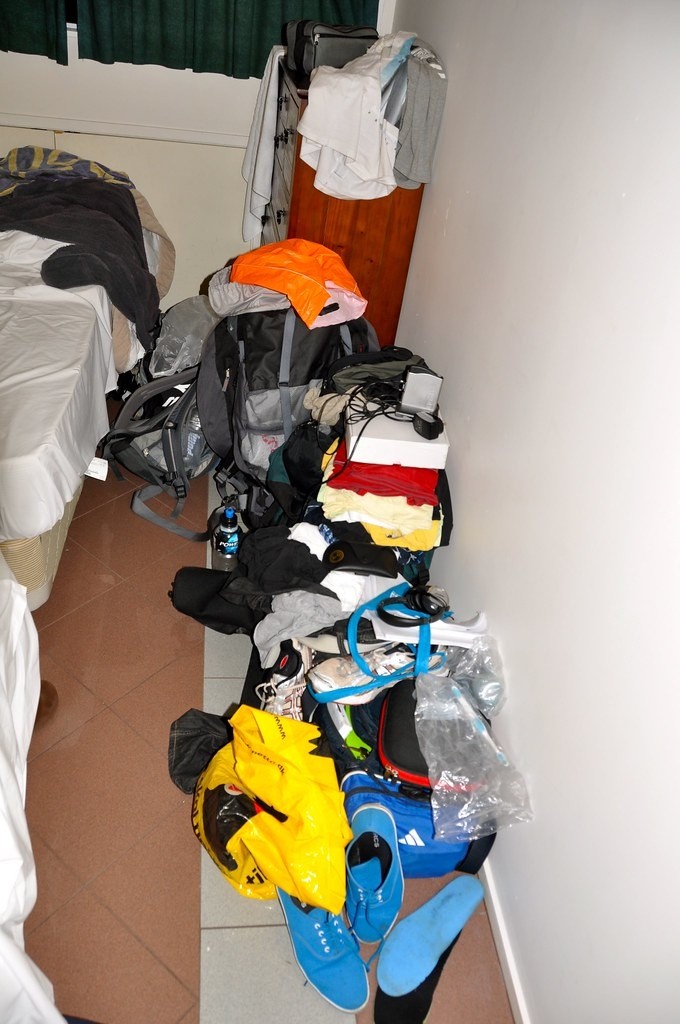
[
  {"xmin": 101, "ymin": 363, "xmax": 223, "ymax": 502},
  {"xmin": 196, "ymin": 307, "xmax": 380, "ymax": 501}
]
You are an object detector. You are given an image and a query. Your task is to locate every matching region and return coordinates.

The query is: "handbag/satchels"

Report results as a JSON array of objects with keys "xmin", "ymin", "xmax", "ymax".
[
  {"xmin": 301, "ymin": 659, "xmax": 492, "ymax": 879},
  {"xmin": 229, "ymin": 237, "xmax": 367, "ymax": 330},
  {"xmin": 192, "ymin": 705, "xmax": 354, "ymax": 915},
  {"xmin": 281, "ymin": 20, "xmax": 379, "ymax": 89}
]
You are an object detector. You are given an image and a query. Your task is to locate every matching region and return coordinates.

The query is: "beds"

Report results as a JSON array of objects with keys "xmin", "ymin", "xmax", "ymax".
[{"xmin": 0, "ymin": 230, "xmax": 115, "ymax": 611}]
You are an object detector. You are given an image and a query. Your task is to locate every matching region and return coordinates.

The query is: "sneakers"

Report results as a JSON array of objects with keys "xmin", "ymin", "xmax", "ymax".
[
  {"xmin": 276, "ymin": 886, "xmax": 370, "ymax": 1013},
  {"xmin": 347, "ymin": 804, "xmax": 405, "ymax": 944}
]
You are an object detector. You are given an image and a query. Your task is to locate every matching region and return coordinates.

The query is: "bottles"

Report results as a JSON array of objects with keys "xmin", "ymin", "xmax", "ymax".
[
  {"xmin": 212, "ymin": 508, "xmax": 243, "ymax": 572},
  {"xmin": 182, "ymin": 406, "xmax": 207, "ymax": 468}
]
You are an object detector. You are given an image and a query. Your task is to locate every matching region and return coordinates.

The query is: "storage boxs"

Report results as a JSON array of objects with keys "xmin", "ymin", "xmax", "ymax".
[{"xmin": 345, "ymin": 400, "xmax": 450, "ymax": 468}]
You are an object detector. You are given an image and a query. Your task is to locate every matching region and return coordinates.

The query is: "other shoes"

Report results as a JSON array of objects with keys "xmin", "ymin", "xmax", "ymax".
[{"xmin": 32, "ymin": 681, "xmax": 58, "ymax": 732}]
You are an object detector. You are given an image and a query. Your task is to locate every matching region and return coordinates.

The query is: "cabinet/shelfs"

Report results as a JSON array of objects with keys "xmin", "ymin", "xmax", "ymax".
[{"xmin": 261, "ymin": 57, "xmax": 425, "ymax": 351}]
